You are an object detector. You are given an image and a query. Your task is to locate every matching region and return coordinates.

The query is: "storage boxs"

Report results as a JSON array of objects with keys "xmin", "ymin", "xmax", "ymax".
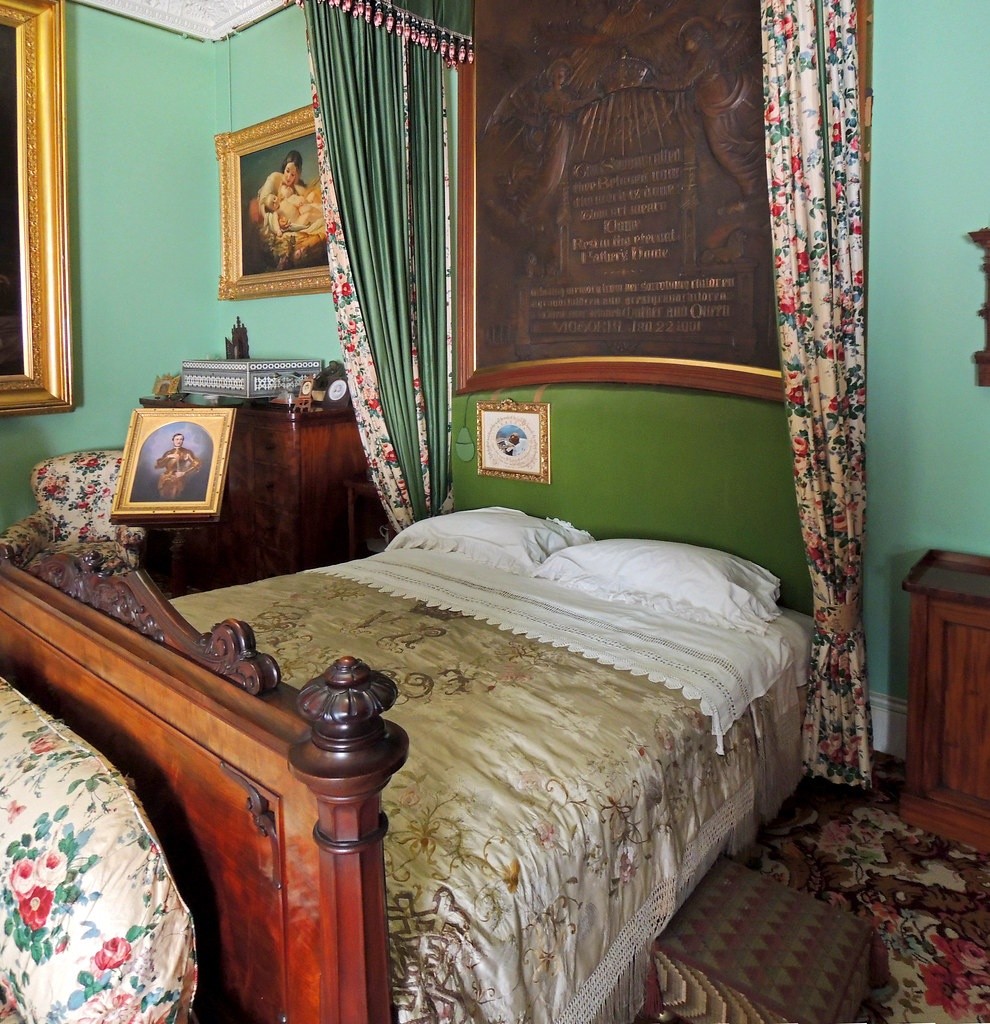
[{"xmin": 180, "ymin": 358, "xmax": 322, "ymax": 397}]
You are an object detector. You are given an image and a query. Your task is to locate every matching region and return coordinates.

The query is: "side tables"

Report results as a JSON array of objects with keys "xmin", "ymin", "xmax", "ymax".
[
  {"xmin": 901, "ymin": 551, "xmax": 990, "ymax": 852},
  {"xmin": 344, "ymin": 471, "xmax": 412, "ymax": 560}
]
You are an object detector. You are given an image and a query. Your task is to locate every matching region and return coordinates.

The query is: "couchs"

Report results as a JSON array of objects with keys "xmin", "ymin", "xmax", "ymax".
[
  {"xmin": 2, "ymin": 444, "xmax": 132, "ymax": 586},
  {"xmin": 1, "ymin": 676, "xmax": 203, "ymax": 1023}
]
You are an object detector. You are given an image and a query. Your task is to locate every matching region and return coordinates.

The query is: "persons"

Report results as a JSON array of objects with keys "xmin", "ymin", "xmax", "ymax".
[{"xmin": 157, "ymin": 433, "xmax": 201, "ymax": 501}]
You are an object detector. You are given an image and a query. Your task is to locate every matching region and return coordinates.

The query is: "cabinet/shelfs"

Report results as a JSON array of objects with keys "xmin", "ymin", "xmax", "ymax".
[{"xmin": 136, "ymin": 398, "xmax": 358, "ymax": 577}]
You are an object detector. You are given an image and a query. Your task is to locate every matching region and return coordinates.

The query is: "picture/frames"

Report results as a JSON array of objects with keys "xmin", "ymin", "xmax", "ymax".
[
  {"xmin": 300, "ymin": 376, "xmax": 311, "ymax": 396},
  {"xmin": 155, "ymin": 376, "xmax": 176, "ymax": 398},
  {"xmin": 324, "ymin": 377, "xmax": 349, "ymax": 408},
  {"xmin": 112, "ymin": 401, "xmax": 234, "ymax": 516},
  {"xmin": 0, "ymin": 0, "xmax": 76, "ymax": 417},
  {"xmin": 476, "ymin": 404, "xmax": 552, "ymax": 484},
  {"xmin": 212, "ymin": 100, "xmax": 340, "ymax": 300}
]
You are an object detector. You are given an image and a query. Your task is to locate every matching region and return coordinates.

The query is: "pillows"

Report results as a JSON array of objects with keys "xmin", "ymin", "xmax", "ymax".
[
  {"xmin": 385, "ymin": 507, "xmax": 598, "ymax": 577},
  {"xmin": 532, "ymin": 539, "xmax": 781, "ymax": 633}
]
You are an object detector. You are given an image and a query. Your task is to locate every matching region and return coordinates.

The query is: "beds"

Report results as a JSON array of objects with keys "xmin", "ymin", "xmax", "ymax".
[
  {"xmin": 451, "ymin": 5, "xmax": 871, "ymax": 397},
  {"xmin": 1, "ymin": 383, "xmax": 816, "ymax": 1023}
]
